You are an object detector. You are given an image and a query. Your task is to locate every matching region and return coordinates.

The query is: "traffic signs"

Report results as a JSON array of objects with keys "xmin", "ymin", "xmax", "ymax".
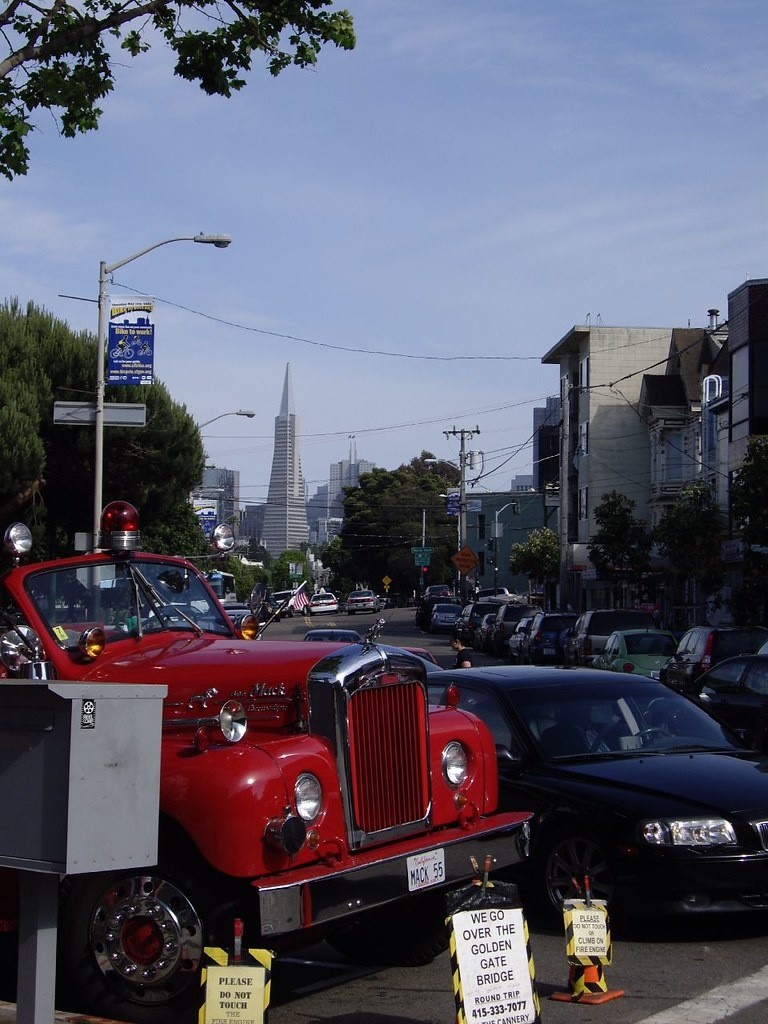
[{"xmin": 412, "ymin": 547, "xmax": 433, "ymax": 567}]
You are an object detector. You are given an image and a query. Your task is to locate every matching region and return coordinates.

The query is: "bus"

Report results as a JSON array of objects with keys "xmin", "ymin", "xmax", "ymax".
[{"xmin": 200, "ymin": 569, "xmax": 237, "ymax": 604}]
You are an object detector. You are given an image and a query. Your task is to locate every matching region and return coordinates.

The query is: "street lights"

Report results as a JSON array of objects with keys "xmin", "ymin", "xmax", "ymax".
[
  {"xmin": 494, "ymin": 503, "xmax": 518, "ymax": 596},
  {"xmin": 439, "ymin": 491, "xmax": 467, "ymax": 602},
  {"xmin": 88, "ymin": 233, "xmax": 231, "ymax": 620}
]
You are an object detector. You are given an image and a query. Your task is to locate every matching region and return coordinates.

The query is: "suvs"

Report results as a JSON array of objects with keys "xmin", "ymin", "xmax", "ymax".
[
  {"xmin": 566, "ymin": 609, "xmax": 679, "ymax": 681},
  {"xmin": 424, "ymin": 585, "xmax": 454, "ymax": 601},
  {"xmin": 272, "ymin": 589, "xmax": 308, "ymax": 618},
  {"xmin": 466, "ymin": 587, "xmax": 519, "ymax": 603}
]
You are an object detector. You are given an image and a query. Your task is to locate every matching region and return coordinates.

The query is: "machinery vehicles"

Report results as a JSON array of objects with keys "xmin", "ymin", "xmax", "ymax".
[{"xmin": 0, "ymin": 502, "xmax": 535, "ymax": 1024}]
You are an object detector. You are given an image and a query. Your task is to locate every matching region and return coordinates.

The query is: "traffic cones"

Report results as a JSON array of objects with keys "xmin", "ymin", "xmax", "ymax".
[{"xmin": 550, "ymin": 965, "xmax": 625, "ymax": 1005}]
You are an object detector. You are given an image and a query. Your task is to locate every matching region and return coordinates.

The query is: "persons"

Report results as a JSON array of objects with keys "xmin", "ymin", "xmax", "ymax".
[{"xmin": 450, "ymin": 632, "xmax": 472, "ymax": 668}]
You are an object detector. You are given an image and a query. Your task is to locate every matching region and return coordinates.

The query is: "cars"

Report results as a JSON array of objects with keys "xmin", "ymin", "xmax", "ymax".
[
  {"xmin": 347, "ymin": 590, "xmax": 380, "ymax": 615},
  {"xmin": 308, "ymin": 587, "xmax": 340, "ymax": 616},
  {"xmin": 415, "ymin": 597, "xmax": 581, "ymax": 666},
  {"xmin": 661, "ymin": 625, "xmax": 768, "ymax": 753},
  {"xmin": 299, "ymin": 629, "xmax": 767, "ymax": 934}
]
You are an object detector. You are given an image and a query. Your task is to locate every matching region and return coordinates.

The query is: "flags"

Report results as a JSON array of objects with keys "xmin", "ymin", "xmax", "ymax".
[{"xmin": 294, "ymin": 585, "xmax": 309, "ymax": 609}]
